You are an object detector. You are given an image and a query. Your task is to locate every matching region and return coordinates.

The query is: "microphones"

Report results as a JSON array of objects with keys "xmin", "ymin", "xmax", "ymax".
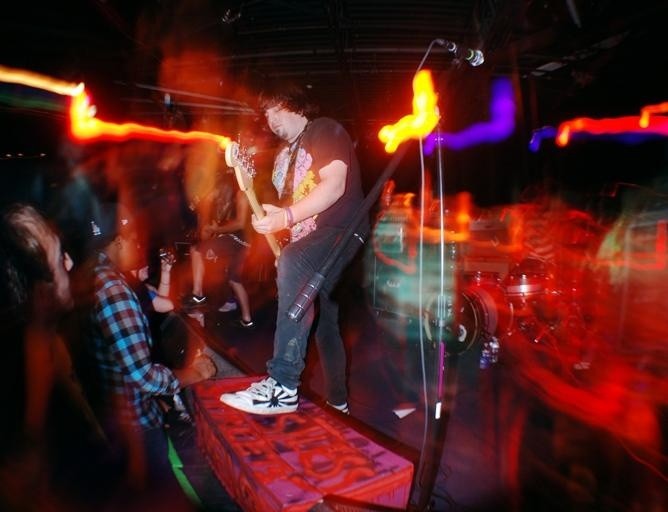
[{"xmin": 444, "ymin": 41, "xmax": 485, "ymax": 67}]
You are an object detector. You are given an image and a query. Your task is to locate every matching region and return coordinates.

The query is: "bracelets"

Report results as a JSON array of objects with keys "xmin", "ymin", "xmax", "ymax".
[{"xmin": 285, "ymin": 204, "xmax": 294, "ymax": 228}]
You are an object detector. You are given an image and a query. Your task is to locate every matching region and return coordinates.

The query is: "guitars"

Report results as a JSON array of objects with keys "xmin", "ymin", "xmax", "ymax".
[{"xmin": 225, "ymin": 134, "xmax": 281, "ymax": 267}]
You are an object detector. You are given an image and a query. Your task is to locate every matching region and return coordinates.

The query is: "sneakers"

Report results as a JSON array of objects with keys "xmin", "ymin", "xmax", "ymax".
[
  {"xmin": 178, "ymin": 293, "xmax": 207, "ymax": 309},
  {"xmin": 219, "ymin": 302, "xmax": 238, "ymax": 312},
  {"xmin": 220, "ymin": 376, "xmax": 299, "ymax": 414},
  {"xmin": 229, "ymin": 318, "xmax": 255, "ymax": 330}
]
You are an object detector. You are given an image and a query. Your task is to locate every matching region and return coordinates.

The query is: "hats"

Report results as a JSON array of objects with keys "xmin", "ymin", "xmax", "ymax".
[{"xmin": 81, "ymin": 204, "xmax": 135, "ymax": 247}]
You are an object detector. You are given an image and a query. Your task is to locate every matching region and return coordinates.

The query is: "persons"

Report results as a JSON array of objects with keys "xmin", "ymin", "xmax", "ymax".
[
  {"xmin": 180, "ymin": 151, "xmax": 256, "ymax": 332},
  {"xmin": 1, "ymin": 197, "xmax": 218, "ymax": 512},
  {"xmin": 217, "ymin": 79, "xmax": 370, "ymax": 419}
]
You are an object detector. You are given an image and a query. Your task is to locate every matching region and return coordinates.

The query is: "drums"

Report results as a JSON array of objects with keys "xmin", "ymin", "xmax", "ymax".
[
  {"xmin": 463, "ymin": 270, "xmax": 500, "ymax": 286},
  {"xmin": 423, "ymin": 282, "xmax": 514, "ymax": 359},
  {"xmin": 506, "ymin": 274, "xmax": 547, "ymax": 317}
]
